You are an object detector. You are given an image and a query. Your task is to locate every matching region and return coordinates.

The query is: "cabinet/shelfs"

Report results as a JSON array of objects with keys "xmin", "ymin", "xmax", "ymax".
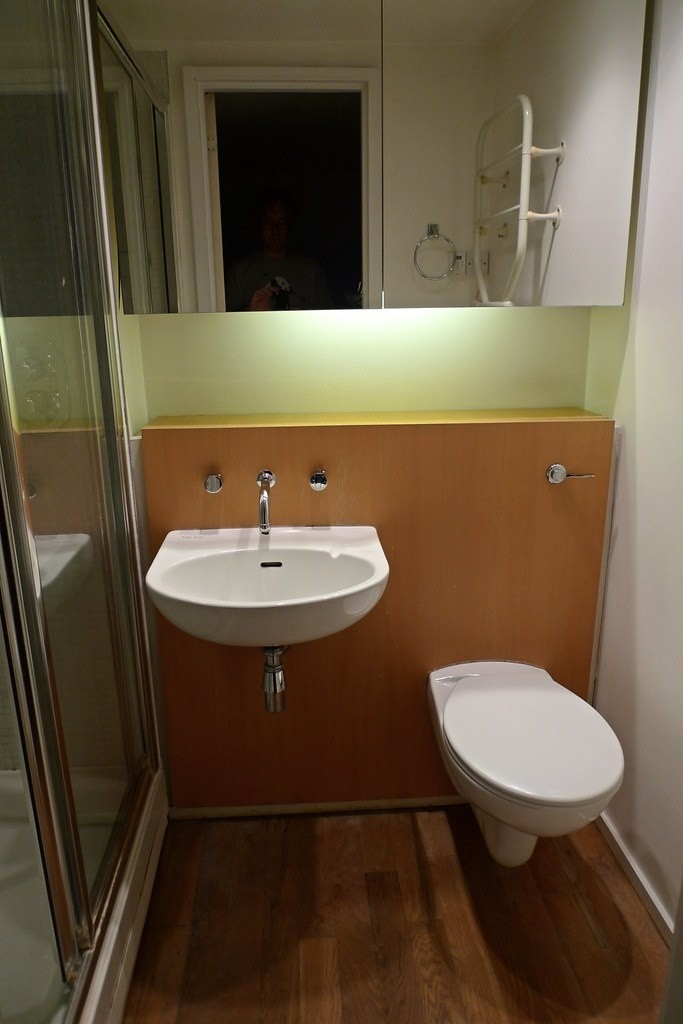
[{"xmin": 474, "ymin": 95, "xmax": 564, "ymax": 307}]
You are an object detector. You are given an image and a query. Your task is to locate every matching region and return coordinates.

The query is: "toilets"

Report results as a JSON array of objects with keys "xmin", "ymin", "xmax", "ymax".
[{"xmin": 426, "ymin": 659, "xmax": 624, "ymax": 868}]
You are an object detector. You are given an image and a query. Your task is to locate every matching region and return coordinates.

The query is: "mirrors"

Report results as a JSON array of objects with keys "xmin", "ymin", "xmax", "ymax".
[{"xmin": 81, "ymin": 0, "xmax": 650, "ymax": 315}]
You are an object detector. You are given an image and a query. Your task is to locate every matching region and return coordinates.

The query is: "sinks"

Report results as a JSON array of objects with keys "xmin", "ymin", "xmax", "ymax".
[{"xmin": 144, "ymin": 548, "xmax": 391, "ymax": 650}]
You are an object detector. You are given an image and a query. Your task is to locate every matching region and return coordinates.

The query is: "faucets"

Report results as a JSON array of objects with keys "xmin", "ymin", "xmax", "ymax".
[{"xmin": 257, "ymin": 470, "xmax": 276, "ymax": 535}]
